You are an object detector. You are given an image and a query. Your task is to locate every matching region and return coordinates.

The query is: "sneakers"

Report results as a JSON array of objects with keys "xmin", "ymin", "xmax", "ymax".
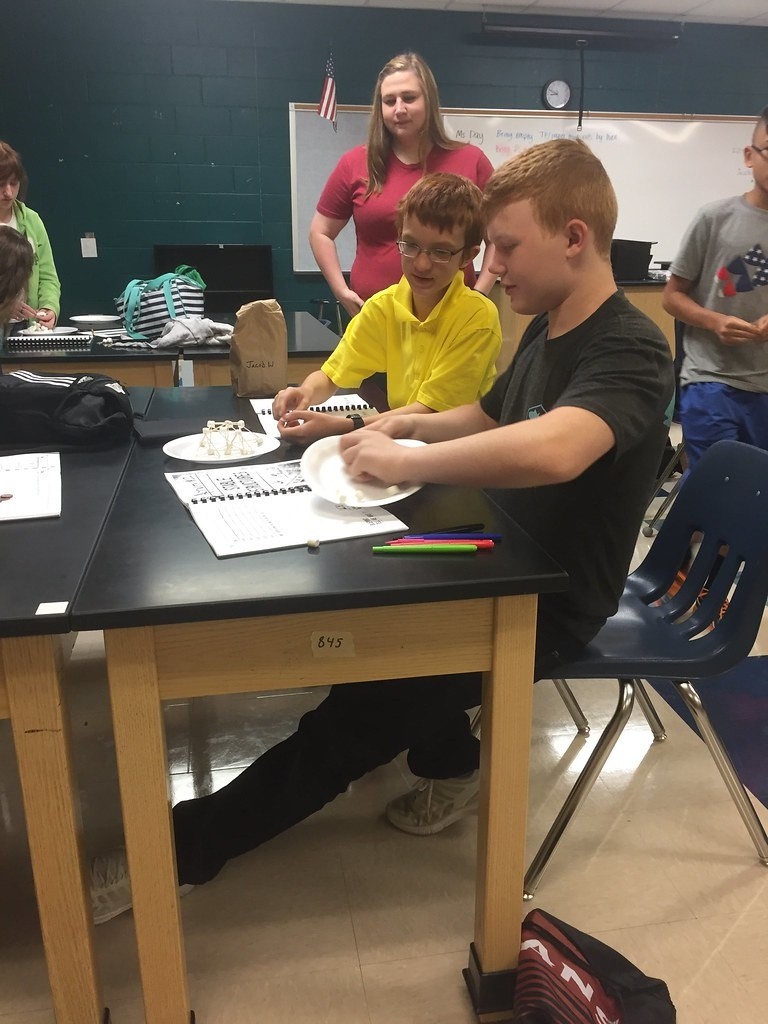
[
  {"xmin": 386, "ymin": 767, "xmax": 481, "ymax": 835},
  {"xmin": 87, "ymin": 844, "xmax": 197, "ymax": 925}
]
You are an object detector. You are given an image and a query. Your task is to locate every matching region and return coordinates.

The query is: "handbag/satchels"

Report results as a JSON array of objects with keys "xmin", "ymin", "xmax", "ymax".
[
  {"xmin": 113, "ymin": 265, "xmax": 205, "ymax": 341},
  {"xmin": 229, "ymin": 299, "xmax": 289, "ymax": 398}
]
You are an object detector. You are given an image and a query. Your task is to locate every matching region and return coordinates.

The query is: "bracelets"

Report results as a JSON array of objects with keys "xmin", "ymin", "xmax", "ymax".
[{"xmin": 346, "ymin": 414, "xmax": 365, "ymax": 428}]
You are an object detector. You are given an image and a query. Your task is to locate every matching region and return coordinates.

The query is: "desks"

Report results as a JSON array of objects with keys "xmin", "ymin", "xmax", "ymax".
[{"xmin": 0, "ymin": 270, "xmax": 675, "ymax": 1024}]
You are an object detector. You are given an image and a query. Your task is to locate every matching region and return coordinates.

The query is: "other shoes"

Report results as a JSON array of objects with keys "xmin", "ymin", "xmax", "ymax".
[{"xmin": 693, "ymin": 589, "xmax": 730, "ymax": 633}]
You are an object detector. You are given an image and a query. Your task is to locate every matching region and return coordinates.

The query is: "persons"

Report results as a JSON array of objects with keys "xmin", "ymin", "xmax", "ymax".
[
  {"xmin": 661, "ymin": 104, "xmax": 768, "ymax": 629},
  {"xmin": 0, "ymin": 140, "xmax": 61, "ymax": 333},
  {"xmin": 274, "ymin": 172, "xmax": 501, "ymax": 446},
  {"xmin": 82, "ymin": 143, "xmax": 675, "ymax": 926},
  {"xmin": 0, "ymin": 225, "xmax": 33, "ymax": 326},
  {"xmin": 308, "ymin": 50, "xmax": 501, "ymax": 317}
]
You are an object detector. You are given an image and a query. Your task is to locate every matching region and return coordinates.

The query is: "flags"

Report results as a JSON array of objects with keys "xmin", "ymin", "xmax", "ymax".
[{"xmin": 317, "ymin": 51, "xmax": 337, "ymax": 132}]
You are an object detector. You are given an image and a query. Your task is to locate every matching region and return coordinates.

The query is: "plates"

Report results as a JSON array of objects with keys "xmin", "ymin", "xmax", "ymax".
[
  {"xmin": 163, "ymin": 431, "xmax": 280, "ymax": 464},
  {"xmin": 18, "ymin": 327, "xmax": 78, "ymax": 336},
  {"xmin": 69, "ymin": 315, "xmax": 120, "ymax": 323},
  {"xmin": 301, "ymin": 435, "xmax": 428, "ymax": 508}
]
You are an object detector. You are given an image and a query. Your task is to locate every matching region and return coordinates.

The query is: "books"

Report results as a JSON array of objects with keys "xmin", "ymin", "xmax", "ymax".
[
  {"xmin": 7, "ymin": 334, "xmax": 93, "ymax": 344},
  {"xmin": 248, "ymin": 394, "xmax": 378, "ymax": 439},
  {"xmin": 161, "ymin": 458, "xmax": 408, "ymax": 558}
]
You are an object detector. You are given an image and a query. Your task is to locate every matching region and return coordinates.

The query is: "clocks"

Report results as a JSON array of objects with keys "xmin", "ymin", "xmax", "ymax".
[{"xmin": 543, "ymin": 79, "xmax": 571, "ymax": 109}]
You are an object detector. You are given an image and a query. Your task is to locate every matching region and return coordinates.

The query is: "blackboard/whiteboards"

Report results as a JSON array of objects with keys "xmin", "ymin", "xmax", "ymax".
[{"xmin": 289, "ymin": 102, "xmax": 761, "ymax": 279}]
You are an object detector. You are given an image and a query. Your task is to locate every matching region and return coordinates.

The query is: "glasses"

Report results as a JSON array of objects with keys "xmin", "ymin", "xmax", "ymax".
[
  {"xmin": 395, "ymin": 239, "xmax": 468, "ymax": 264},
  {"xmin": 752, "ymin": 145, "xmax": 768, "ymax": 160}
]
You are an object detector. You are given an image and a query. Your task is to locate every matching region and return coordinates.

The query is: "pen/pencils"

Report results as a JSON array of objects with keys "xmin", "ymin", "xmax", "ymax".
[{"xmin": 373, "ymin": 522, "xmax": 503, "ymax": 553}]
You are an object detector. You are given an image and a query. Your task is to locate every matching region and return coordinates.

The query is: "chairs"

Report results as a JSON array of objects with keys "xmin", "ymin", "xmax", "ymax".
[{"xmin": 468, "ymin": 439, "xmax": 768, "ymax": 900}]
